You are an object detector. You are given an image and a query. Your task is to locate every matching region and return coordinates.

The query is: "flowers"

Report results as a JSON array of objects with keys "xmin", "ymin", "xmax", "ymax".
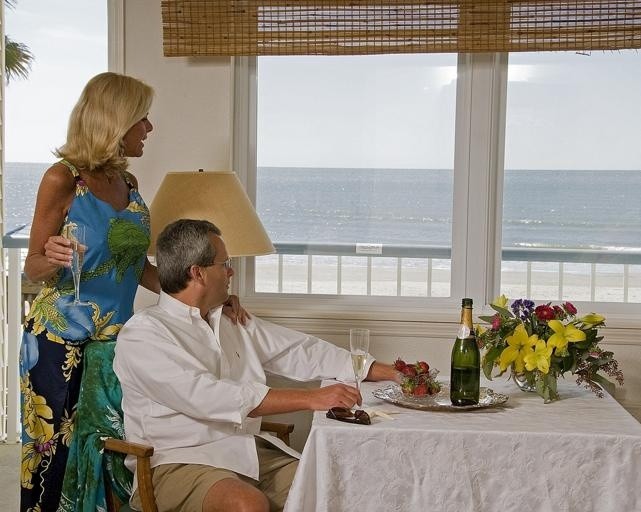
[{"xmin": 473, "ymin": 295, "xmax": 625, "ymax": 404}]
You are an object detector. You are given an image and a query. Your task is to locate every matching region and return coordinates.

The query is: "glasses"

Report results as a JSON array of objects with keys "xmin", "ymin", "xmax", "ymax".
[
  {"xmin": 199, "ymin": 257, "xmax": 232, "ymax": 270},
  {"xmin": 326, "ymin": 407, "xmax": 370, "ymax": 425}
]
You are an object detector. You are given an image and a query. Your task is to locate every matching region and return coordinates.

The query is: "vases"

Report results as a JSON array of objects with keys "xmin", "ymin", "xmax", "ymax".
[{"xmin": 511, "ymin": 365, "xmax": 536, "ymax": 393}]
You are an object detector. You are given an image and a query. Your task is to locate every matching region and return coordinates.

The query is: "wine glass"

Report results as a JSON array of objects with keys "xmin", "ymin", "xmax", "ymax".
[
  {"xmin": 66, "ymin": 224, "xmax": 92, "ymax": 307},
  {"xmin": 350, "ymin": 328, "xmax": 370, "ymax": 410}
]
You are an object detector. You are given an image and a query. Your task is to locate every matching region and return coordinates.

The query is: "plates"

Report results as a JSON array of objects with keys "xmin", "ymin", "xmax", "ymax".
[{"xmin": 372, "ymin": 380, "xmax": 512, "ymax": 414}]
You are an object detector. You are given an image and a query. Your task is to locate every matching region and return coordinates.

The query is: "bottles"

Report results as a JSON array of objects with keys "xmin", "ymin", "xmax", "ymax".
[{"xmin": 451, "ymin": 298, "xmax": 481, "ymax": 404}]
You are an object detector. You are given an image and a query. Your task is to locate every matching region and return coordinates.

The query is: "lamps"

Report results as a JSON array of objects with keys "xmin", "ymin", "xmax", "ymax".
[{"xmin": 147, "ymin": 169, "xmax": 276, "ymax": 258}]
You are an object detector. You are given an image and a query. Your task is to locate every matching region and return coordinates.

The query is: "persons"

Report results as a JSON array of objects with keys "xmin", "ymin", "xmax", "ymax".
[
  {"xmin": 22, "ymin": 73, "xmax": 254, "ymax": 509},
  {"xmin": 116, "ymin": 219, "xmax": 410, "ymax": 511}
]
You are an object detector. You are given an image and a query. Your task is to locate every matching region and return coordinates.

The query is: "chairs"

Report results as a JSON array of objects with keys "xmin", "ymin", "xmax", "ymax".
[{"xmin": 82, "ymin": 340, "xmax": 295, "ymax": 512}]
